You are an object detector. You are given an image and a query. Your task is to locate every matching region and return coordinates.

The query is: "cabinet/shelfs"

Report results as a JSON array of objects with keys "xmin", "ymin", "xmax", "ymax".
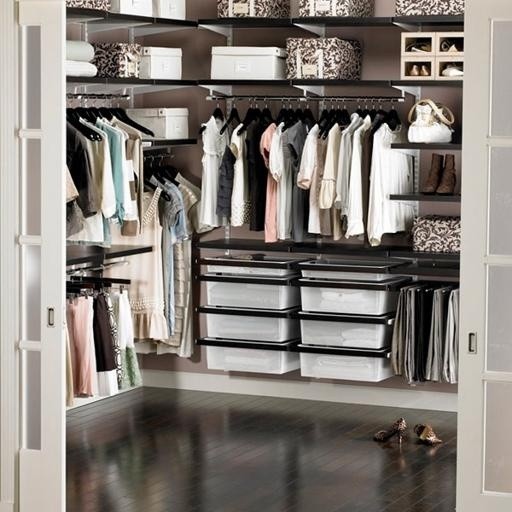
[
  {"xmin": 199, "ymin": 17, "xmax": 465, "ymax": 414},
  {"xmin": 67, "ymin": 5, "xmax": 201, "ymax": 409}
]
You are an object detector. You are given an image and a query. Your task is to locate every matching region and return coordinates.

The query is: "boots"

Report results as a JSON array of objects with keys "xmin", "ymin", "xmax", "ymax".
[{"xmin": 422, "ymin": 152, "xmax": 457, "ymax": 195}]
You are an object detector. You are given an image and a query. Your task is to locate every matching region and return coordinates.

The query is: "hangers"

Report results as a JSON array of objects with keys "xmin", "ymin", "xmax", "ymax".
[
  {"xmin": 143, "ymin": 150, "xmax": 203, "ymax": 201},
  {"xmin": 69, "ymin": 92, "xmax": 156, "ymax": 143},
  {"xmin": 213, "ymin": 94, "xmax": 402, "ymax": 128},
  {"xmin": 66, "ymin": 264, "xmax": 132, "ymax": 300}
]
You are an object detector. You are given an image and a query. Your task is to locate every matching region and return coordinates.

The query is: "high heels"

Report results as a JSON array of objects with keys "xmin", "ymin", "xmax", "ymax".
[{"xmin": 373, "ymin": 419, "xmax": 442, "ymax": 448}]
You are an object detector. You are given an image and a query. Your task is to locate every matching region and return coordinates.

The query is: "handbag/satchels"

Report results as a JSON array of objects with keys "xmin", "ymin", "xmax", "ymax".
[{"xmin": 407, "ymin": 98, "xmax": 455, "ymax": 143}]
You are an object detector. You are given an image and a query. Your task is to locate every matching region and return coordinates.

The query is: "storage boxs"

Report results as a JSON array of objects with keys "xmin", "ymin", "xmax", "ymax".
[
  {"xmin": 90, "ymin": 40, "xmax": 141, "ymax": 80},
  {"xmin": 67, "ymin": 0, "xmax": 188, "ymax": 22},
  {"xmin": 204, "ymin": 256, "xmax": 411, "ymax": 382},
  {"xmin": 210, "ymin": 45, "xmax": 286, "ymax": 81},
  {"xmin": 393, "ymin": 0, "xmax": 465, "ymax": 18},
  {"xmin": 216, "ymin": 0, "xmax": 290, "ymax": 19},
  {"xmin": 139, "ymin": 46, "xmax": 183, "ymax": 82},
  {"xmin": 297, "ymin": 0, "xmax": 376, "ymax": 19},
  {"xmin": 125, "ymin": 107, "xmax": 189, "ymax": 141},
  {"xmin": 285, "ymin": 36, "xmax": 361, "ymax": 80}
]
